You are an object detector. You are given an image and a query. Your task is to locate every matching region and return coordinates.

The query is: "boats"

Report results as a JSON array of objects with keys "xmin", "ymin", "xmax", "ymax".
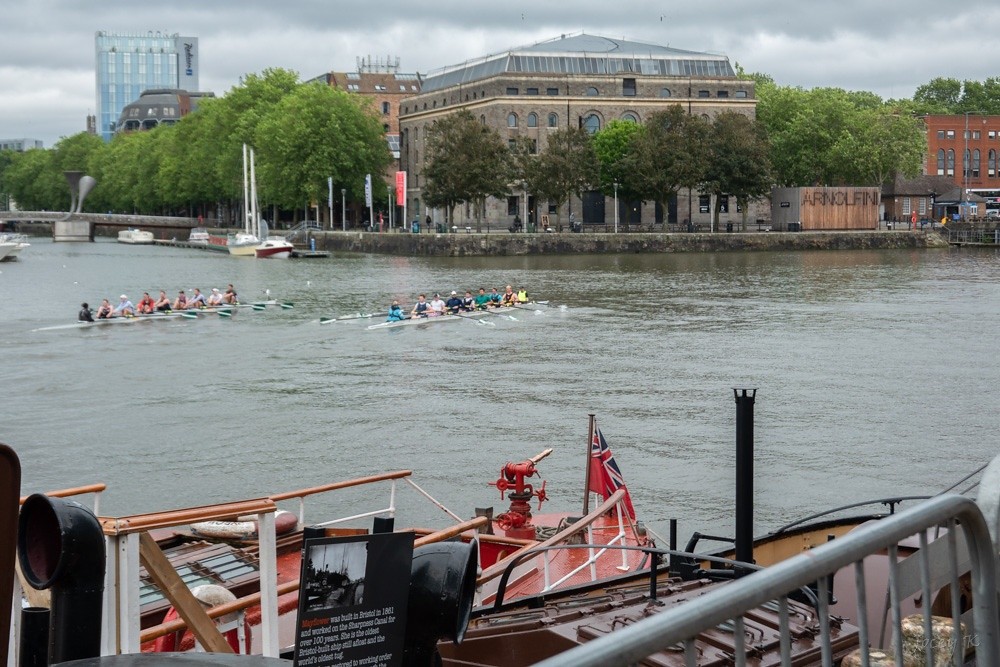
[
  {"xmin": 254, "ymin": 236, "xmax": 294, "ymax": 260},
  {"xmin": 118, "ymin": 230, "xmax": 154, "ymax": 244},
  {"xmin": 369, "ymin": 300, "xmax": 550, "ymax": 328},
  {"xmin": 226, "ymin": 233, "xmax": 260, "ymax": 256},
  {"xmin": 0, "ymin": 233, "xmax": 31, "ymax": 262},
  {"xmin": 139, "ymin": 410, "xmax": 659, "ymax": 654},
  {"xmin": 189, "ymin": 227, "xmax": 210, "ymax": 243},
  {"xmin": 276, "ymin": 462, "xmax": 988, "ymax": 667},
  {"xmin": 33, "ymin": 299, "xmax": 280, "ymax": 332}
]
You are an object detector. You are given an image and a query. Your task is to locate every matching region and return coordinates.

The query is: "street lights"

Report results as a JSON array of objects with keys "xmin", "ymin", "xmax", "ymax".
[
  {"xmin": 613, "ymin": 177, "xmax": 618, "ymax": 234},
  {"xmin": 965, "ymin": 112, "xmax": 987, "ymax": 187}
]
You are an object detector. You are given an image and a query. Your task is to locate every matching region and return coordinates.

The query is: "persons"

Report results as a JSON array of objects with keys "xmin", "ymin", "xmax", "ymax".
[
  {"xmin": 96, "ymin": 299, "xmax": 113, "ymax": 318},
  {"xmin": 172, "ymin": 290, "xmax": 189, "ymax": 310},
  {"xmin": 410, "ymin": 295, "xmax": 434, "ymax": 319},
  {"xmin": 459, "ymin": 291, "xmax": 475, "ymax": 312},
  {"xmin": 223, "ymin": 284, "xmax": 238, "ymax": 305},
  {"xmin": 500, "ymin": 285, "xmax": 518, "ymax": 307},
  {"xmin": 155, "ymin": 291, "xmax": 171, "ymax": 312},
  {"xmin": 426, "ymin": 293, "xmax": 445, "ymax": 317},
  {"xmin": 514, "ymin": 214, "xmax": 522, "ymax": 233},
  {"xmin": 445, "ymin": 291, "xmax": 463, "ymax": 315},
  {"xmin": 568, "ymin": 213, "xmax": 574, "ymax": 230},
  {"xmin": 487, "ymin": 288, "xmax": 503, "ymax": 308},
  {"xmin": 113, "ymin": 294, "xmax": 134, "ymax": 317},
  {"xmin": 207, "ymin": 288, "xmax": 223, "ymax": 306},
  {"xmin": 386, "ymin": 300, "xmax": 405, "ymax": 321},
  {"xmin": 138, "ymin": 292, "xmax": 154, "ymax": 314},
  {"xmin": 188, "ymin": 288, "xmax": 207, "ymax": 309},
  {"xmin": 518, "ymin": 286, "xmax": 529, "ymax": 304},
  {"xmin": 79, "ymin": 303, "xmax": 94, "ymax": 322},
  {"xmin": 426, "ymin": 214, "xmax": 431, "ymax": 233},
  {"xmin": 475, "ymin": 288, "xmax": 491, "ymax": 310},
  {"xmin": 528, "ymin": 209, "xmax": 536, "ymax": 228}
]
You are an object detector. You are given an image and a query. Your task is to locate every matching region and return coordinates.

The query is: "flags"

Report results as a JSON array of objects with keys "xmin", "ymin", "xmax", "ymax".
[{"xmin": 589, "ymin": 424, "xmax": 637, "ymax": 523}]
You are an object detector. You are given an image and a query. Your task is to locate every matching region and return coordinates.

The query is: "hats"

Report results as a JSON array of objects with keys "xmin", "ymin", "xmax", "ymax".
[
  {"xmin": 119, "ymin": 295, "xmax": 127, "ymax": 299},
  {"xmin": 433, "ymin": 292, "xmax": 440, "ymax": 298},
  {"xmin": 178, "ymin": 291, "xmax": 185, "ymax": 293},
  {"xmin": 392, "ymin": 301, "xmax": 400, "ymax": 306},
  {"xmin": 451, "ymin": 291, "xmax": 456, "ymax": 295},
  {"xmin": 212, "ymin": 288, "xmax": 219, "ymax": 291}
]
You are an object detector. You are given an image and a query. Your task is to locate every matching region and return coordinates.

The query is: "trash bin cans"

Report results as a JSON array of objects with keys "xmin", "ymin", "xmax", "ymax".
[
  {"xmin": 788, "ymin": 222, "xmax": 799, "ymax": 231},
  {"xmin": 727, "ymin": 220, "xmax": 733, "ymax": 232},
  {"xmin": 526, "ymin": 222, "xmax": 535, "ymax": 233},
  {"xmin": 436, "ymin": 223, "xmax": 448, "ymax": 233},
  {"xmin": 364, "ymin": 221, "xmax": 369, "ymax": 231}
]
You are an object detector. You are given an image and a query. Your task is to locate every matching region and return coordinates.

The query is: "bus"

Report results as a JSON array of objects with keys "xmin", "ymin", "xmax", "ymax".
[{"xmin": 966, "ymin": 188, "xmax": 1000, "ymax": 203}]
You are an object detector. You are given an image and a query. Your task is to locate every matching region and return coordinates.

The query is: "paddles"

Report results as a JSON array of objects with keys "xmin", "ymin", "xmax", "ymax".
[
  {"xmin": 93, "ymin": 300, "xmax": 295, "ymax": 319},
  {"xmin": 320, "ymin": 301, "xmax": 567, "ymax": 326}
]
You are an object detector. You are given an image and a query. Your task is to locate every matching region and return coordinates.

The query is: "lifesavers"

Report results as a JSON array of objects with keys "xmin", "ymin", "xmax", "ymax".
[
  {"xmin": 154, "ymin": 584, "xmax": 249, "ymax": 653},
  {"xmin": 189, "ymin": 510, "xmax": 299, "ymax": 539},
  {"xmin": 912, "ymin": 217, "xmax": 916, "ymax": 223}
]
